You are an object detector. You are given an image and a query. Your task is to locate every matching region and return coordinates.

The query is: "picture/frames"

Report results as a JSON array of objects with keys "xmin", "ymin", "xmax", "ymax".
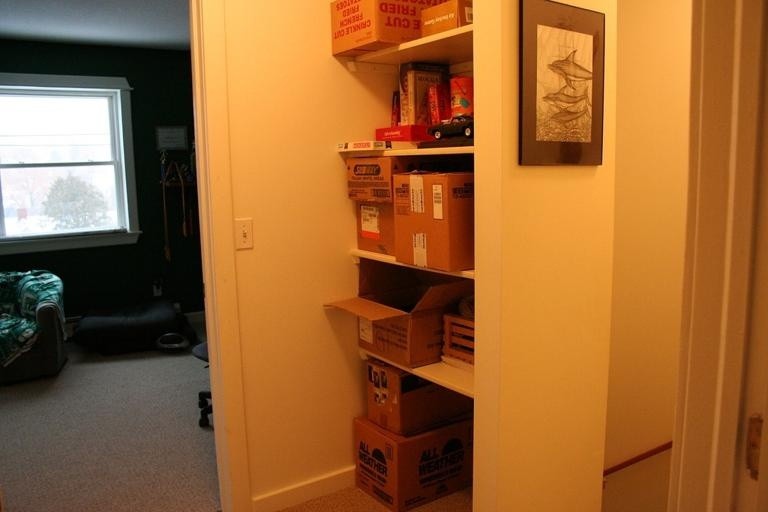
[{"xmin": 518, "ymin": 0, "xmax": 606, "ymax": 167}]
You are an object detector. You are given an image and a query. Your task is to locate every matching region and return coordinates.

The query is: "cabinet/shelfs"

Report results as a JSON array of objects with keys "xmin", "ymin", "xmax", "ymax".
[{"xmin": 350, "ymin": 25, "xmax": 475, "ymax": 402}]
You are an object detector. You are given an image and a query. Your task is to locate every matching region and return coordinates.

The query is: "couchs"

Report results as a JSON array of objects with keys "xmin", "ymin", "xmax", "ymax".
[{"xmin": 0, "ymin": 268, "xmax": 69, "ymax": 387}]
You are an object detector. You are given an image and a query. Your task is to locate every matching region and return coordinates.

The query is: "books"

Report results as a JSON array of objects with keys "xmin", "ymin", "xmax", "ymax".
[{"xmin": 339, "ymin": 65, "xmax": 445, "ymax": 153}]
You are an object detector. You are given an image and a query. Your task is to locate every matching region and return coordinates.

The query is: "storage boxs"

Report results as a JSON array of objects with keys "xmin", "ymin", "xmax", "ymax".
[
  {"xmin": 419, "ymin": 0, "xmax": 471, "ymax": 39},
  {"xmin": 366, "ymin": 357, "xmax": 474, "ymax": 434},
  {"xmin": 346, "ymin": 157, "xmax": 425, "ymax": 204},
  {"xmin": 353, "ymin": 417, "xmax": 473, "ymax": 511},
  {"xmin": 331, "ymin": 0, "xmax": 446, "ymax": 58},
  {"xmin": 322, "ymin": 259, "xmax": 473, "ymax": 370},
  {"xmin": 391, "ymin": 171, "xmax": 474, "ymax": 273},
  {"xmin": 354, "ymin": 200, "xmax": 394, "ymax": 256}
]
